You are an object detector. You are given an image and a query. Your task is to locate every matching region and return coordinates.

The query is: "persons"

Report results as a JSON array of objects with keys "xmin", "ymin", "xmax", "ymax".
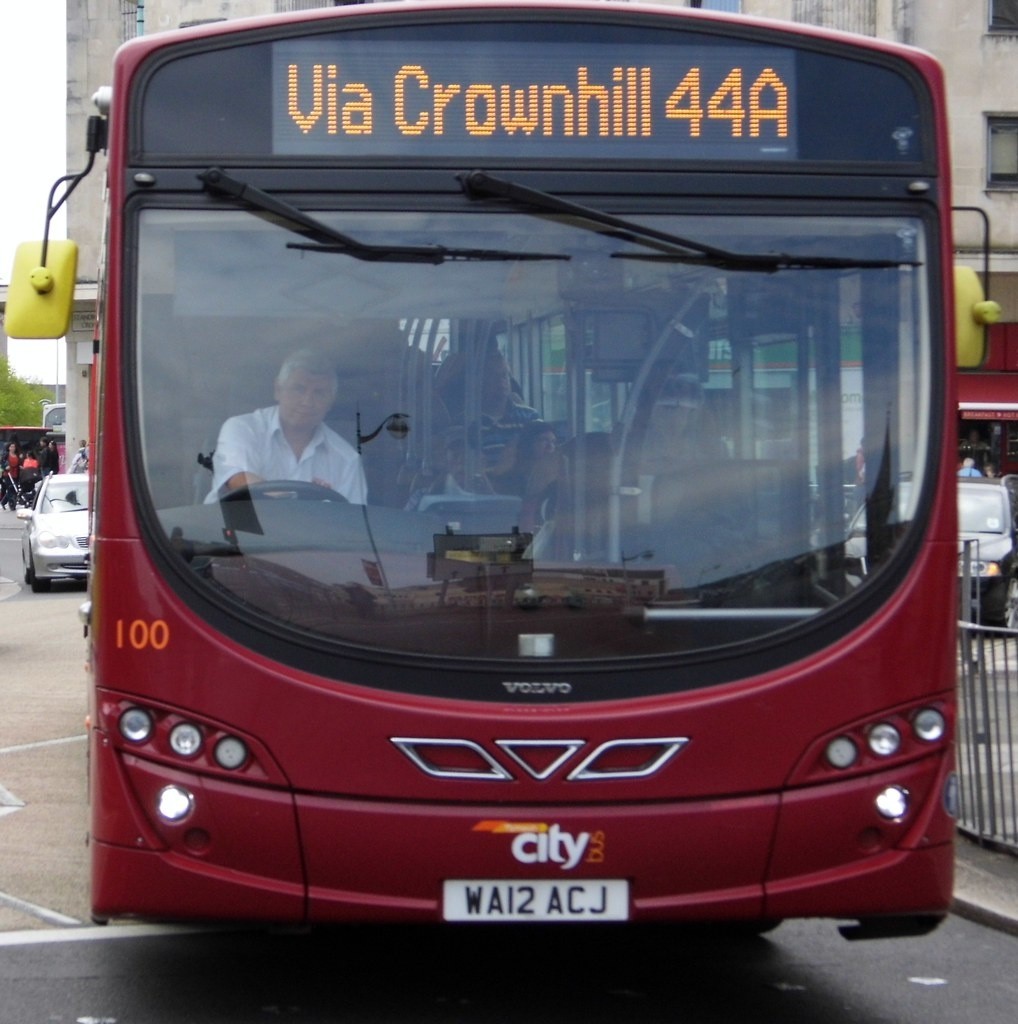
[
  {"xmin": 983, "ymin": 463, "xmax": 1003, "ymax": 478},
  {"xmin": 199, "ymin": 347, "xmax": 369, "ymax": 509},
  {"xmin": 844, "ymin": 438, "xmax": 864, "ymax": 485},
  {"xmin": 0, "ymin": 437, "xmax": 90, "ymax": 510},
  {"xmin": 957, "ymin": 457, "xmax": 981, "ymax": 478},
  {"xmin": 339, "ymin": 319, "xmax": 719, "ymax": 569}
]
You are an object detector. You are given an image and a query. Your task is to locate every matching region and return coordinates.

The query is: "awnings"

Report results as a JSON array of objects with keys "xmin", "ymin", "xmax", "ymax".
[{"xmin": 956, "ymin": 373, "xmax": 1018, "ymax": 420}]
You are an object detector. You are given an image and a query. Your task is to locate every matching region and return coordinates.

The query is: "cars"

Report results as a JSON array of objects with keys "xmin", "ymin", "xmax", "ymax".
[
  {"xmin": 16, "ymin": 470, "xmax": 95, "ymax": 592},
  {"xmin": 844, "ymin": 475, "xmax": 1018, "ymax": 634}
]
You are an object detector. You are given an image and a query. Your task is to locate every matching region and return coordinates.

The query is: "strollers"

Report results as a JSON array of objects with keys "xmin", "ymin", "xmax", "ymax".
[{"xmin": 6, "ymin": 465, "xmax": 40, "ymax": 506}]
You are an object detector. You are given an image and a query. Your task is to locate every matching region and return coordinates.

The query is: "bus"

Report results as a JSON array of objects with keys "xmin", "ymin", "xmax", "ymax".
[
  {"xmin": 4, "ymin": 0, "xmax": 1003, "ymax": 943},
  {"xmin": 0, "ymin": 426, "xmax": 55, "ymax": 501}
]
